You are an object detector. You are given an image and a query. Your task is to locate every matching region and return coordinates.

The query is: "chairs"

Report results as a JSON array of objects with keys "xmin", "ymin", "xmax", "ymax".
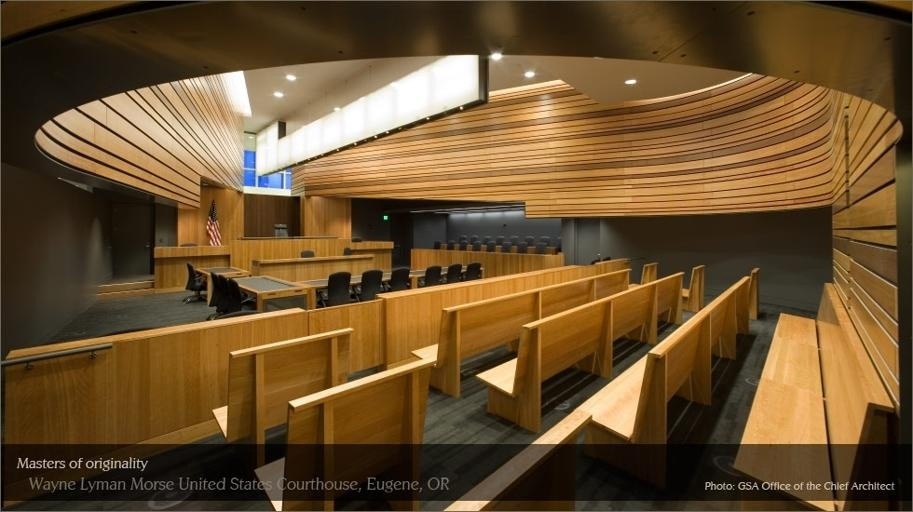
[
  {"xmin": 181, "ymin": 243, "xmax": 197, "ymax": 246},
  {"xmin": 301, "ymin": 250, "xmax": 314, "ymax": 257},
  {"xmin": 273, "ymin": 223, "xmax": 288, "ymax": 237},
  {"xmin": 344, "ymin": 247, "xmax": 351, "ymax": 256},
  {"xmin": 352, "ymin": 237, "xmax": 362, "ymax": 242},
  {"xmin": 430, "ymin": 231, "xmax": 564, "ymax": 251},
  {"xmin": 184, "ymin": 255, "xmax": 487, "ymax": 317}
]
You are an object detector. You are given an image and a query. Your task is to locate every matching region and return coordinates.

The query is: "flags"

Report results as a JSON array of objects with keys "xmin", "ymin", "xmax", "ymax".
[{"xmin": 206, "ymin": 199, "xmax": 222, "ymax": 246}]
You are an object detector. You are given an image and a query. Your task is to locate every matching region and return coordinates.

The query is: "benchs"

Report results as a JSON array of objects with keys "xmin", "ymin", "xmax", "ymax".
[
  {"xmin": 733, "ymin": 282, "xmax": 895, "ymax": 512},
  {"xmin": 209, "ymin": 257, "xmax": 769, "ymax": 512}
]
